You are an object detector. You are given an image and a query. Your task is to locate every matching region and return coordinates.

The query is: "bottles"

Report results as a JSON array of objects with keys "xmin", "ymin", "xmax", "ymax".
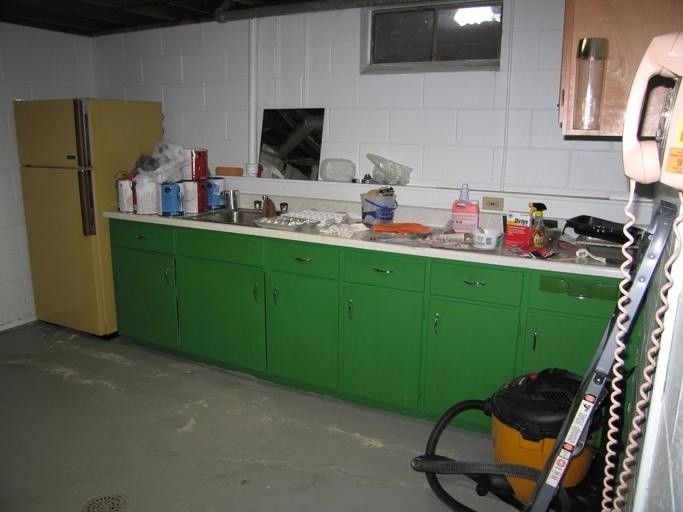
[
  {"xmin": 577, "ymin": 38, "xmax": 606, "ymax": 131},
  {"xmin": 460, "ymin": 183, "xmax": 469, "ymax": 206}
]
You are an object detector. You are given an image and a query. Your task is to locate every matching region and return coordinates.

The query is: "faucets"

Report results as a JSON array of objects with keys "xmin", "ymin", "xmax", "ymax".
[{"xmin": 262, "ymin": 195, "xmax": 269, "ymax": 202}]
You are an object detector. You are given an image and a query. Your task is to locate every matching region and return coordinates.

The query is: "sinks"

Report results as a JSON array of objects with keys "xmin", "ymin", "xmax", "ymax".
[{"xmin": 180, "ymin": 208, "xmax": 280, "ymax": 227}]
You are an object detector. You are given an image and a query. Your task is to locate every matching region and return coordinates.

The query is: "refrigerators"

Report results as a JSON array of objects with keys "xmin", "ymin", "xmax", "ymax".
[{"xmin": 16, "ymin": 97, "xmax": 165, "ymax": 338}]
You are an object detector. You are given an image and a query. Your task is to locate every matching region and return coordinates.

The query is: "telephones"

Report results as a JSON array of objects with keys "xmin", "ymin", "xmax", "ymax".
[{"xmin": 622, "ymin": 32, "xmax": 683, "ymax": 193}]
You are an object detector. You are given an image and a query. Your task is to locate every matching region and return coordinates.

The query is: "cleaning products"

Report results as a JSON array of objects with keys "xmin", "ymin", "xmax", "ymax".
[{"xmin": 529, "ymin": 202, "xmax": 547, "ymax": 248}]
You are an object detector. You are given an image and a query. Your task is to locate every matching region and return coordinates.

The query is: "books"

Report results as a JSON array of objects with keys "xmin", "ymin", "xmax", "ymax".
[{"xmin": 503, "ymin": 210, "xmax": 531, "ymax": 249}]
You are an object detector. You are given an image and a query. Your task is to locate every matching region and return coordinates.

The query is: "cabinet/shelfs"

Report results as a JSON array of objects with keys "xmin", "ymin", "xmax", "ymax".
[
  {"xmin": 520, "ymin": 272, "xmax": 618, "ymax": 446},
  {"xmin": 556, "ymin": 1, "xmax": 683, "ymax": 138},
  {"xmin": 176, "ymin": 228, "xmax": 266, "ymax": 375},
  {"xmin": 338, "ymin": 250, "xmax": 429, "ymax": 409},
  {"xmin": 266, "ymin": 243, "xmax": 339, "ymax": 390},
  {"xmin": 425, "ymin": 261, "xmax": 523, "ymax": 427},
  {"xmin": 109, "ymin": 218, "xmax": 175, "ymax": 347}
]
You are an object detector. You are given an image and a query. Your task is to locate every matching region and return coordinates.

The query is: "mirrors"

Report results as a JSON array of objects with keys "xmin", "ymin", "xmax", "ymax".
[{"xmin": 256, "ymin": 107, "xmax": 325, "ymax": 181}]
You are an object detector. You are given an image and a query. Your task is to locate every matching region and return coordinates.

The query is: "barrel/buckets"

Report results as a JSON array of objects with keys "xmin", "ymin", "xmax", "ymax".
[
  {"xmin": 452, "ymin": 200, "xmax": 479, "ymax": 232},
  {"xmin": 361, "ymin": 194, "xmax": 399, "ymax": 226}
]
